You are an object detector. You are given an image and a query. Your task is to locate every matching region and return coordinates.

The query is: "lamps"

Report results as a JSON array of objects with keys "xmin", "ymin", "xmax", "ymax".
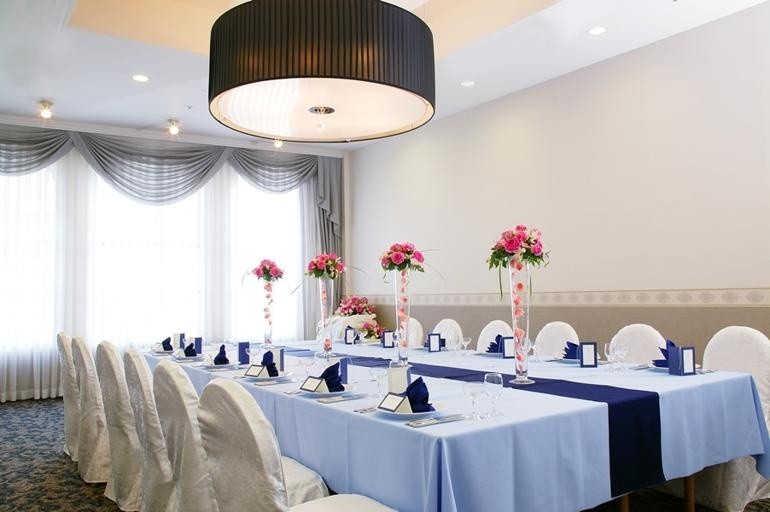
[
  {"xmin": 168, "ymin": 119, "xmax": 179, "ymax": 134},
  {"xmin": 40, "ymin": 100, "xmax": 53, "ymax": 119},
  {"xmin": 208, "ymin": 0, "xmax": 436, "ymax": 143}
]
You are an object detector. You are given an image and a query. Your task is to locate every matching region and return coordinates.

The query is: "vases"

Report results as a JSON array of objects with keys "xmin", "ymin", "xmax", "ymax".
[
  {"xmin": 507, "ymin": 256, "xmax": 535, "ymax": 385},
  {"xmin": 260, "ymin": 282, "xmax": 274, "ymax": 348},
  {"xmin": 317, "ymin": 272, "xmax": 336, "ymax": 358},
  {"xmin": 395, "ymin": 266, "xmax": 411, "ymax": 369}
]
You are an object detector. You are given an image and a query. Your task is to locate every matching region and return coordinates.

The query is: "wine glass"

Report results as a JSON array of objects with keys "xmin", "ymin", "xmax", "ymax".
[
  {"xmin": 357, "ymin": 329, "xmax": 367, "ymax": 346},
  {"xmin": 349, "ymin": 332, "xmax": 357, "ymax": 348},
  {"xmin": 604, "ymin": 343, "xmax": 616, "ymax": 375},
  {"xmin": 246, "ymin": 349, "xmax": 257, "ymax": 365},
  {"xmin": 299, "ymin": 357, "xmax": 314, "ymax": 379},
  {"xmin": 484, "ymin": 373, "xmax": 504, "ymax": 417},
  {"xmin": 316, "ymin": 349, "xmax": 326, "ymax": 376},
  {"xmin": 259, "ymin": 344, "xmax": 267, "ymax": 360},
  {"xmin": 461, "ymin": 338, "xmax": 471, "ymax": 356},
  {"xmin": 465, "ymin": 381, "xmax": 482, "ymax": 419},
  {"xmin": 369, "ymin": 364, "xmax": 390, "ymax": 400},
  {"xmin": 375, "ymin": 402, "xmax": 441, "ymax": 416},
  {"xmin": 615, "ymin": 337, "xmax": 628, "ymax": 373},
  {"xmin": 303, "ymin": 383, "xmax": 353, "ymax": 396},
  {"xmin": 450, "ymin": 335, "xmax": 459, "ymax": 357},
  {"xmin": 224, "ymin": 339, "xmax": 233, "ymax": 361},
  {"xmin": 390, "ymin": 361, "xmax": 405, "ymax": 394},
  {"xmin": 392, "ymin": 331, "xmax": 398, "ymax": 350}
]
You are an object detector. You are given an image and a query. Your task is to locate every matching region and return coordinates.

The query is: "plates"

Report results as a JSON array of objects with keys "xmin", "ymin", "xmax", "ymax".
[
  {"xmin": 408, "ymin": 345, "xmax": 425, "ymax": 350},
  {"xmin": 648, "ymin": 362, "xmax": 700, "ymax": 370},
  {"xmin": 175, "ymin": 354, "xmax": 204, "ymax": 359},
  {"xmin": 556, "ymin": 355, "xmax": 601, "ymax": 363},
  {"xmin": 204, "ymin": 360, "xmax": 239, "ymax": 369},
  {"xmin": 473, "ymin": 350, "xmax": 504, "ymax": 357},
  {"xmin": 243, "ymin": 368, "xmax": 288, "ymax": 379},
  {"xmin": 153, "ymin": 350, "xmax": 173, "ymax": 353},
  {"xmin": 334, "ymin": 339, "xmax": 345, "ymax": 343}
]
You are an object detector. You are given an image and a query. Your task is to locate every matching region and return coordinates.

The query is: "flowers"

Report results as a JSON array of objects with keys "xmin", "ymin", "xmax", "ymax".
[
  {"xmin": 304, "ymin": 253, "xmax": 346, "ymax": 280},
  {"xmin": 488, "ymin": 223, "xmax": 550, "ymax": 301},
  {"xmin": 246, "ymin": 259, "xmax": 286, "ymax": 281},
  {"xmin": 334, "ymin": 295, "xmax": 376, "ymax": 316},
  {"xmin": 379, "ymin": 242, "xmax": 425, "ymax": 283}
]
